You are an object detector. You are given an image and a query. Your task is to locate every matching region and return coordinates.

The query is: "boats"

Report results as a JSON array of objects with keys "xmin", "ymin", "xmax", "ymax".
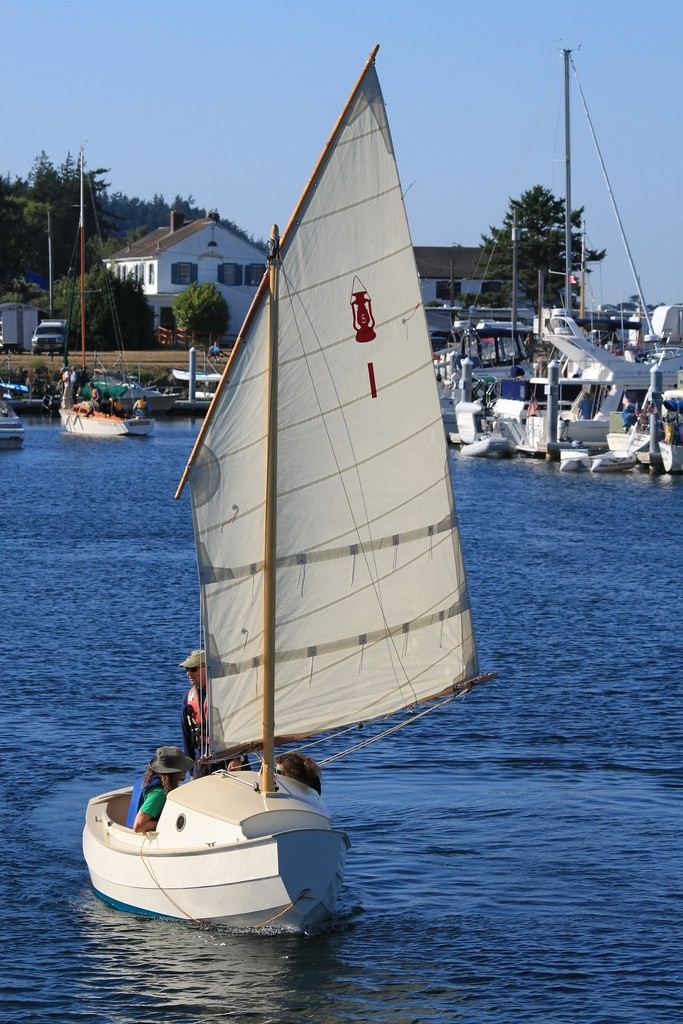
[
  {"xmin": 159, "ymin": 369, "xmax": 225, "ymax": 410},
  {"xmin": 435, "ymin": 39, "xmax": 683, "ymax": 476},
  {"xmin": 76, "ymin": 348, "xmax": 181, "ymax": 414},
  {"xmin": 0, "ymin": 381, "xmax": 30, "ymax": 448}
]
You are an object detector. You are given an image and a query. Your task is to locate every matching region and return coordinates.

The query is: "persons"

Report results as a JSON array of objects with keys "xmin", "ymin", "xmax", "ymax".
[
  {"xmin": 620, "ymin": 398, "xmax": 637, "ymax": 427},
  {"xmin": 127, "ymin": 746, "xmax": 194, "ymax": 833},
  {"xmin": 524, "ymin": 333, "xmax": 533, "ymax": 363},
  {"xmin": 208, "ymin": 341, "xmax": 220, "ymax": 363},
  {"xmin": 97, "ymin": 392, "xmax": 113, "ymax": 414},
  {"xmin": 178, "ymin": 649, "xmax": 252, "ymax": 780},
  {"xmin": 133, "ymin": 395, "xmax": 152, "ymax": 417},
  {"xmin": 60, "ymin": 367, "xmax": 71, "ymax": 409},
  {"xmin": 275, "ymin": 754, "xmax": 321, "ymax": 786},
  {"xmin": 88, "ymin": 382, "xmax": 101, "ymax": 403},
  {"xmin": 70, "ymin": 366, "xmax": 80, "ymax": 404},
  {"xmin": 576, "ymin": 392, "xmax": 592, "ymax": 420}
]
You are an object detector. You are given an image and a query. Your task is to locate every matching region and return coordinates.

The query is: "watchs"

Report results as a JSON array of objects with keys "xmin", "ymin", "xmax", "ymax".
[{"xmin": 240, "ymin": 756, "xmax": 244, "ymax": 762}]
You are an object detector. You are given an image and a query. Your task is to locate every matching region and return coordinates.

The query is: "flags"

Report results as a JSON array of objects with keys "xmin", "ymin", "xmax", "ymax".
[
  {"xmin": 646, "ymin": 400, "xmax": 657, "ymax": 414},
  {"xmin": 527, "ymin": 395, "xmax": 538, "ymax": 416},
  {"xmin": 635, "ymin": 405, "xmax": 646, "ymax": 424}
]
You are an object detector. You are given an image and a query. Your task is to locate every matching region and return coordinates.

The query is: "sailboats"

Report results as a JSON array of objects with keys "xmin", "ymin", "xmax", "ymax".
[
  {"xmin": 58, "ymin": 144, "xmax": 158, "ymax": 438},
  {"xmin": 82, "ymin": 45, "xmax": 498, "ymax": 933}
]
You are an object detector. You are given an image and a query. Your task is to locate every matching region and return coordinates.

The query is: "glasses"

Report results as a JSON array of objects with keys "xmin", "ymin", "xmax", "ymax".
[
  {"xmin": 186, "ymin": 666, "xmax": 200, "ymax": 672},
  {"xmin": 274, "ymin": 769, "xmax": 282, "ymax": 774}
]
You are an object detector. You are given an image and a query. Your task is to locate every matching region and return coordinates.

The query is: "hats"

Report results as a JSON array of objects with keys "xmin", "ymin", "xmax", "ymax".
[
  {"xmin": 149, "ymin": 745, "xmax": 194, "ymax": 773},
  {"xmin": 179, "ymin": 650, "xmax": 205, "ymax": 668}
]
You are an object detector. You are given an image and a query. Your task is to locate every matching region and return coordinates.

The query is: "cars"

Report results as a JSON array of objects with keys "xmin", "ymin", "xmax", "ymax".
[{"xmin": 30, "ymin": 321, "xmax": 67, "ymax": 356}]
